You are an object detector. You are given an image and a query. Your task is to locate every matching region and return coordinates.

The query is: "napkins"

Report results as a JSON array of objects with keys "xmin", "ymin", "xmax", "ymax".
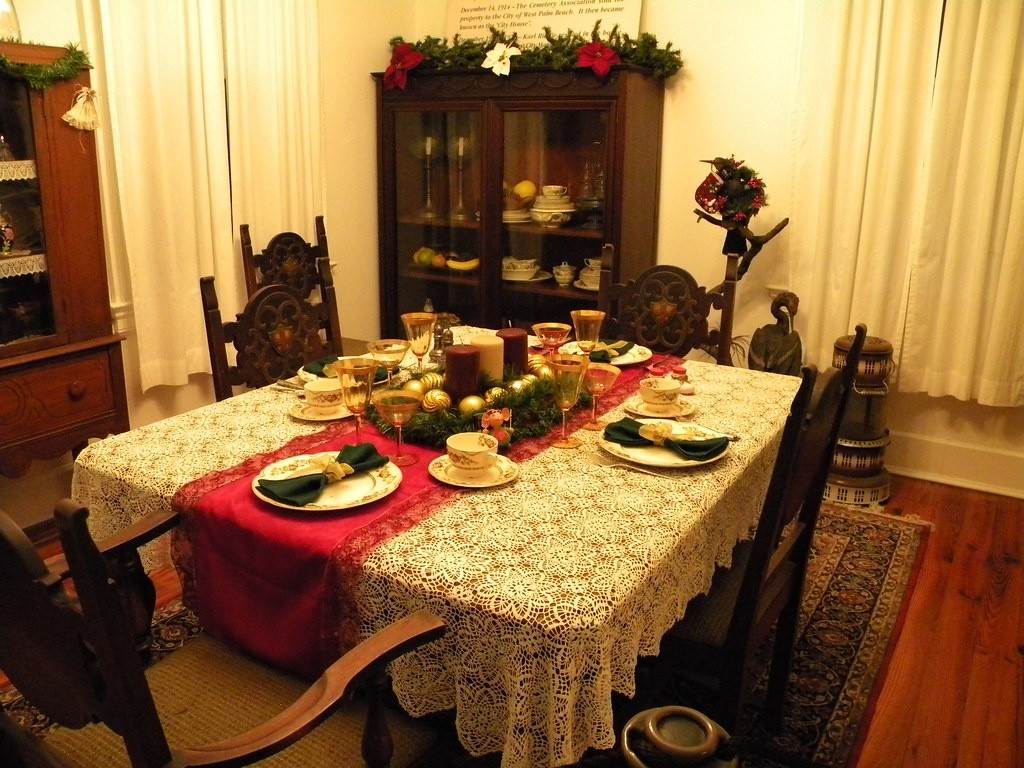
[
  {"xmin": 255, "ymin": 439, "xmax": 389, "ymax": 505},
  {"xmin": 588, "ymin": 339, "xmax": 637, "ymax": 367},
  {"xmin": 303, "ymin": 352, "xmax": 398, "ymax": 380},
  {"xmin": 601, "ymin": 417, "xmax": 729, "ymax": 461}
]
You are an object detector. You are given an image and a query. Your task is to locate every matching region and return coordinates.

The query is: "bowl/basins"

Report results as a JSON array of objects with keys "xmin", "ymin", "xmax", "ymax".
[
  {"xmin": 529, "ymin": 195, "xmax": 576, "ymax": 228},
  {"xmin": 501, "ymin": 266, "xmax": 541, "ymax": 280}
]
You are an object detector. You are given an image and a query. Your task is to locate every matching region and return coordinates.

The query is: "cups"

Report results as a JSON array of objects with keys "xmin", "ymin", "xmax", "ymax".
[
  {"xmin": 553, "ymin": 262, "xmax": 576, "ymax": 286},
  {"xmin": 584, "ymin": 258, "xmax": 601, "ymax": 272},
  {"xmin": 446, "ymin": 432, "xmax": 498, "ymax": 477},
  {"xmin": 543, "ymin": 186, "xmax": 568, "ymax": 199},
  {"xmin": 296, "ymin": 379, "xmax": 342, "ymax": 415},
  {"xmin": 639, "ymin": 377, "xmax": 682, "ymax": 412}
]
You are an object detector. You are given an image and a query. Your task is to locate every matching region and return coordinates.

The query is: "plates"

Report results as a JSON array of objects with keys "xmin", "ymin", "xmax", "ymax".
[
  {"xmin": 298, "ymin": 356, "xmax": 402, "ymax": 386},
  {"xmin": 251, "ymin": 451, "xmax": 403, "ymax": 511},
  {"xmin": 597, "ymin": 419, "xmax": 730, "ymax": 467},
  {"xmin": 623, "ymin": 398, "xmax": 694, "ymax": 418},
  {"xmin": 505, "ymin": 270, "xmax": 552, "ymax": 283},
  {"xmin": 474, "ymin": 208, "xmax": 532, "ymax": 223},
  {"xmin": 428, "ymin": 455, "xmax": 518, "ymax": 488},
  {"xmin": 289, "ymin": 402, "xmax": 354, "ymax": 421},
  {"xmin": 558, "ymin": 340, "xmax": 653, "ymax": 365},
  {"xmin": 572, "ymin": 269, "xmax": 600, "ymax": 292}
]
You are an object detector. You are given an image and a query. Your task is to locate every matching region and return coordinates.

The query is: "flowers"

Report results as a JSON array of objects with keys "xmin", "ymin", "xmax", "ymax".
[{"xmin": 694, "ymin": 156, "xmax": 770, "ymax": 227}]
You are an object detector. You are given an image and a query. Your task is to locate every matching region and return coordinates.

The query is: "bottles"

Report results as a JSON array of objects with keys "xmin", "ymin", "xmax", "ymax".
[
  {"xmin": 646, "ymin": 368, "xmax": 688, "ymax": 384},
  {"xmin": 0, "ymin": 203, "xmax": 15, "ymax": 256}
]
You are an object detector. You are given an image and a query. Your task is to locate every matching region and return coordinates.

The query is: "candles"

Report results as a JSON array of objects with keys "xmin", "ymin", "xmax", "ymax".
[
  {"xmin": 425, "ymin": 136, "xmax": 433, "ymax": 155},
  {"xmin": 444, "ymin": 321, "xmax": 528, "ymax": 399},
  {"xmin": 458, "ymin": 137, "xmax": 464, "ymax": 159}
]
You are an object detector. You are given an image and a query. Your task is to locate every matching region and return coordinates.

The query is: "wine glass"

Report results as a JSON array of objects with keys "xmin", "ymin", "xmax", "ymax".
[{"xmin": 332, "ymin": 308, "xmax": 621, "ymax": 467}]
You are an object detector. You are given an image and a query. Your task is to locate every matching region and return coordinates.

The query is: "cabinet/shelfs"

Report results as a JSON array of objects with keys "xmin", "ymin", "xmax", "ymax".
[
  {"xmin": 0, "ymin": 41, "xmax": 130, "ymax": 478},
  {"xmin": 369, "ymin": 65, "xmax": 665, "ymax": 336}
]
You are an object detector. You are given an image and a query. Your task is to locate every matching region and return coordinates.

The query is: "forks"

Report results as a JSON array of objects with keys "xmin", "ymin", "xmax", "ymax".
[{"xmin": 585, "ymin": 450, "xmax": 675, "ymax": 480}]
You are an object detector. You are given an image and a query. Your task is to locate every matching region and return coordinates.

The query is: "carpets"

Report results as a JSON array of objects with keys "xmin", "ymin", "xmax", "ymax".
[{"xmin": 0, "ymin": 496, "xmax": 933, "ymax": 768}]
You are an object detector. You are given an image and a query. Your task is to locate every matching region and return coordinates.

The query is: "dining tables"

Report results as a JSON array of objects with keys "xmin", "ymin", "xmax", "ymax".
[{"xmin": 70, "ymin": 331, "xmax": 809, "ymax": 767}]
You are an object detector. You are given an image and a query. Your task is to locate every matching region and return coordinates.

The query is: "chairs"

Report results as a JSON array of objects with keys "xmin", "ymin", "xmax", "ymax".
[
  {"xmin": 0, "ymin": 496, "xmax": 446, "ymax": 768},
  {"xmin": 597, "ymin": 244, "xmax": 739, "ymax": 369},
  {"xmin": 200, "ymin": 214, "xmax": 345, "ymax": 401},
  {"xmin": 616, "ymin": 322, "xmax": 868, "ymax": 730}
]
